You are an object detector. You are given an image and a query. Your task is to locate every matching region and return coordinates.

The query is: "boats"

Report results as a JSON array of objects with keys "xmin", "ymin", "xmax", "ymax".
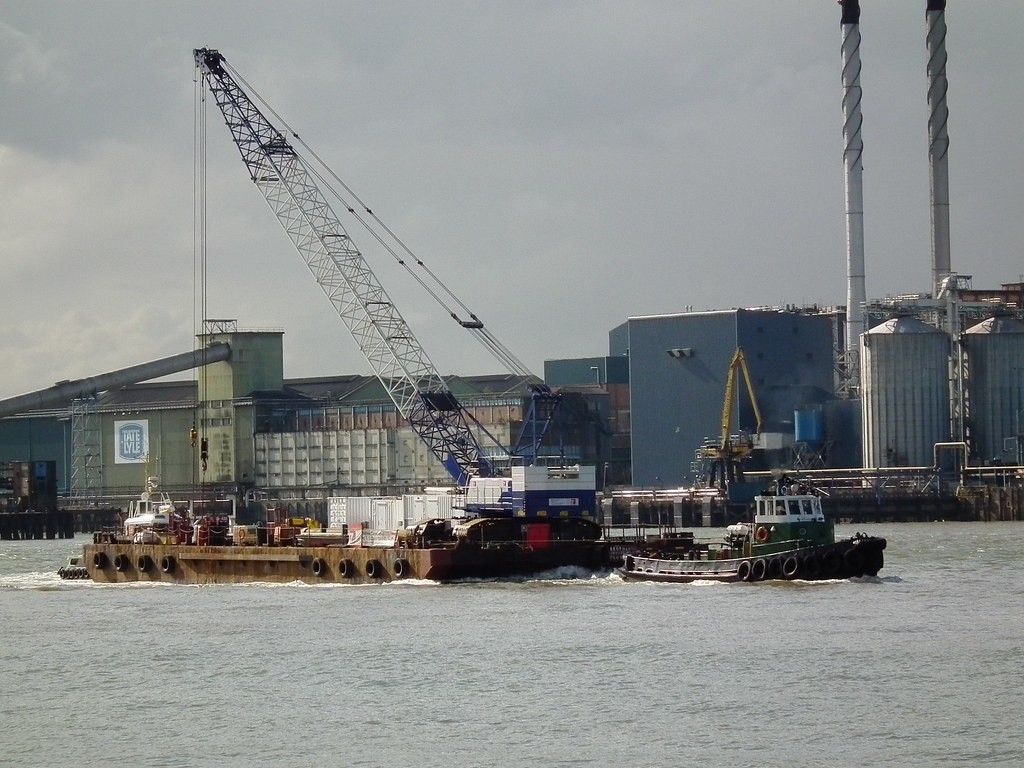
[
  {"xmin": 613, "ymin": 471, "xmax": 886, "ymax": 586},
  {"xmin": 124, "ymin": 475, "xmax": 176, "ymax": 534}
]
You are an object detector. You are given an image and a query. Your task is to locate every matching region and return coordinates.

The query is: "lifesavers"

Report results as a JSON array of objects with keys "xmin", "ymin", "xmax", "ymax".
[
  {"xmin": 113, "ymin": 553, "xmax": 128, "ymax": 571},
  {"xmin": 736, "ymin": 550, "xmax": 840, "ymax": 581},
  {"xmin": 755, "ymin": 525, "xmax": 770, "ymax": 542},
  {"xmin": 93, "ymin": 552, "xmax": 104, "ymax": 568},
  {"xmin": 366, "ymin": 559, "xmax": 381, "ymax": 578},
  {"xmin": 338, "ymin": 558, "xmax": 354, "ymax": 578},
  {"xmin": 160, "ymin": 555, "xmax": 176, "ymax": 573},
  {"xmin": 57, "ymin": 568, "xmax": 89, "ymax": 580},
  {"xmin": 238, "ymin": 529, "xmax": 245, "ymax": 540},
  {"xmin": 311, "ymin": 557, "xmax": 325, "ymax": 576},
  {"xmin": 393, "ymin": 558, "xmax": 409, "ymax": 578},
  {"xmin": 138, "ymin": 555, "xmax": 152, "ymax": 572}
]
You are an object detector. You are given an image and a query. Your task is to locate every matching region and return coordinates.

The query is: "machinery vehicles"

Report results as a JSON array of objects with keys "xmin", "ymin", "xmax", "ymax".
[
  {"xmin": 189, "ymin": 47, "xmax": 615, "ymax": 545},
  {"xmin": 694, "ymin": 346, "xmax": 764, "ymax": 491}
]
